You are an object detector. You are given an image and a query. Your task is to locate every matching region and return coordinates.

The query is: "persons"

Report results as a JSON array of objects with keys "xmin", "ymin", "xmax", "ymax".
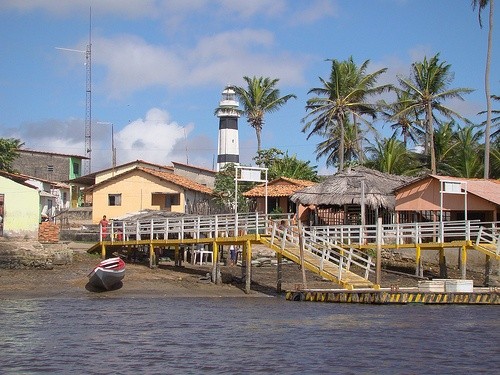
[
  {"xmin": 228, "ymin": 244, "xmax": 238, "ymax": 263},
  {"xmin": 99, "ymin": 215, "xmax": 110, "ymax": 241}
]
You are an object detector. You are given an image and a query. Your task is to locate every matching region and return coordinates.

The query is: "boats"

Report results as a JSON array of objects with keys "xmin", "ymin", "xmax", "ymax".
[{"xmin": 87, "ymin": 256, "xmax": 125, "ymax": 291}]
